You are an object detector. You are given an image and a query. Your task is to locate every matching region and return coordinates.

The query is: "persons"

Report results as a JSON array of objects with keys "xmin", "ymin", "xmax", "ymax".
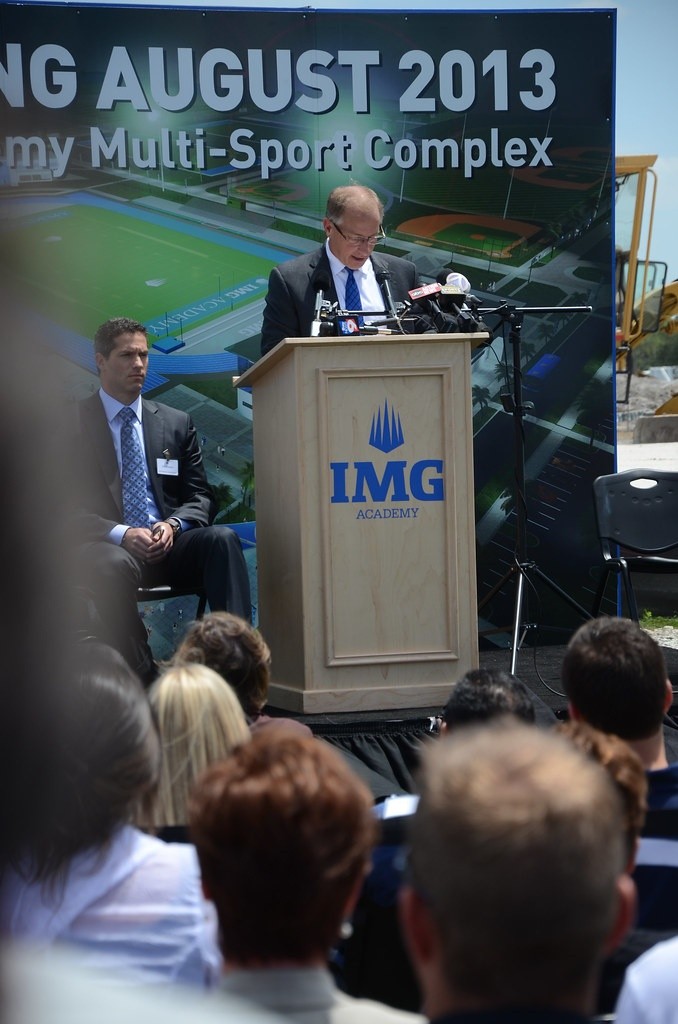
[
  {"xmin": 0, "ymin": 610, "xmax": 678, "ymax": 1024},
  {"xmin": 75, "ymin": 315, "xmax": 253, "ymax": 688},
  {"xmin": 260, "ymin": 184, "xmax": 438, "ymax": 356}
]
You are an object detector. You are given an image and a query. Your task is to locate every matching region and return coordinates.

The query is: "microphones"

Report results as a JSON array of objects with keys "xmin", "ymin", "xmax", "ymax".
[
  {"xmin": 320, "ymin": 315, "xmax": 398, "ymax": 337},
  {"xmin": 376, "ymin": 268, "xmax": 397, "ymax": 317},
  {"xmin": 408, "ymin": 268, "xmax": 483, "ymax": 333}
]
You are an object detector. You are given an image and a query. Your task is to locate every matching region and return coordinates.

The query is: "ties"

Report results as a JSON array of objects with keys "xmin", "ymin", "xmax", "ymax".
[
  {"xmin": 344, "ymin": 266, "xmax": 365, "ymax": 327},
  {"xmin": 116, "ymin": 407, "xmax": 152, "ymax": 528}
]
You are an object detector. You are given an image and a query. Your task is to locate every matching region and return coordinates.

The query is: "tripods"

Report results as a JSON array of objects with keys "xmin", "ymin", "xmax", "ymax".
[{"xmin": 478, "ymin": 305, "xmax": 597, "ymax": 677}]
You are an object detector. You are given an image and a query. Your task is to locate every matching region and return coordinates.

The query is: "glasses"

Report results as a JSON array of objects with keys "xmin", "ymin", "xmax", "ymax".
[{"xmin": 330, "ymin": 220, "xmax": 386, "ymax": 246}]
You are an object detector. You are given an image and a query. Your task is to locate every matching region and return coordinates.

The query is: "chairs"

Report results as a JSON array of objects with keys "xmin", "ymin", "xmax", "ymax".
[
  {"xmin": 594, "ymin": 468, "xmax": 678, "ymax": 618},
  {"xmin": 136, "ymin": 576, "xmax": 207, "ymax": 621}
]
